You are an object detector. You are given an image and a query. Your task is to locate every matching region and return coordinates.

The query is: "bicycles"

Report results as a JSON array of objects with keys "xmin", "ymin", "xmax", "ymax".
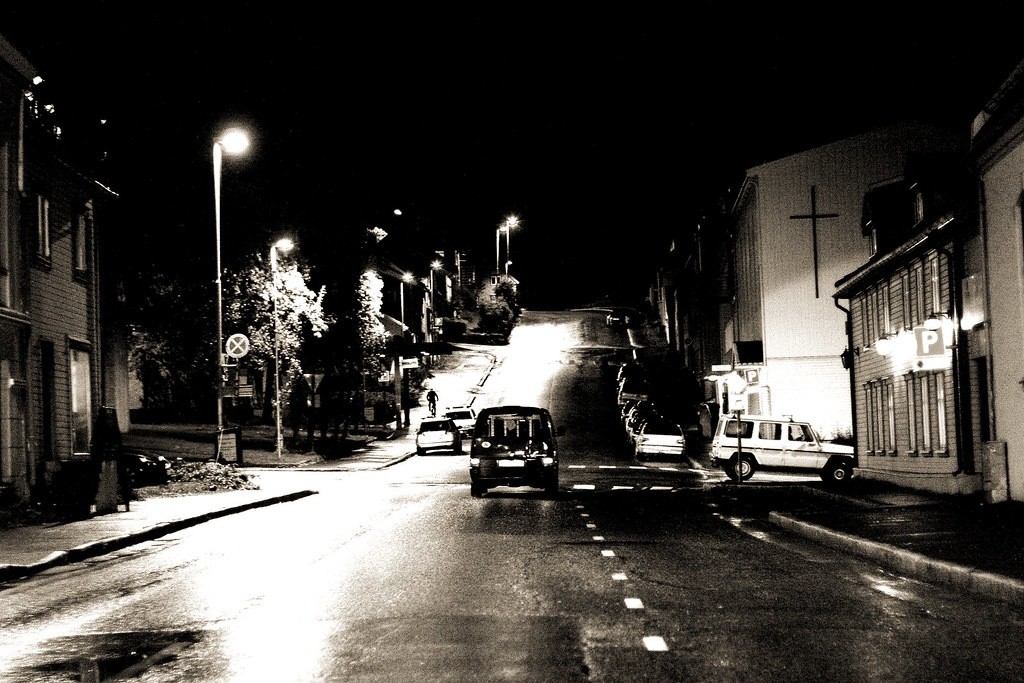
[{"xmin": 428, "ymin": 398, "xmax": 439, "ymax": 417}]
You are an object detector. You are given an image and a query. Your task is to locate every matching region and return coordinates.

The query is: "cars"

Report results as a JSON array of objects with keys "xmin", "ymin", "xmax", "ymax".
[
  {"xmin": 415, "ymin": 416, "xmax": 462, "ymax": 455},
  {"xmin": 634, "ymin": 421, "xmax": 686, "ymax": 461},
  {"xmin": 617, "ymin": 362, "xmax": 663, "ymax": 441},
  {"xmin": 123, "ymin": 447, "xmax": 172, "ymax": 487},
  {"xmin": 441, "ymin": 404, "xmax": 477, "ymax": 438}
]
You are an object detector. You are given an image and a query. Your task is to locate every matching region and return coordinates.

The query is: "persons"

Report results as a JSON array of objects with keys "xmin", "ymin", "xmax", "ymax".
[{"xmin": 426, "ymin": 389, "xmax": 439, "ymax": 417}]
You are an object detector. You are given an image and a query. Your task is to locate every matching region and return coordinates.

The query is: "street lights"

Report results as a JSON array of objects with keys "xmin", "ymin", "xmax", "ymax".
[
  {"xmin": 496, "ymin": 226, "xmax": 507, "ymax": 274},
  {"xmin": 270, "ymin": 234, "xmax": 295, "ymax": 457},
  {"xmin": 211, "ymin": 122, "xmax": 256, "ymax": 430},
  {"xmin": 725, "ymin": 371, "xmax": 745, "ymax": 482},
  {"xmin": 507, "ymin": 212, "xmax": 525, "ymax": 274},
  {"xmin": 430, "ymin": 258, "xmax": 445, "ymax": 337},
  {"xmin": 400, "ymin": 270, "xmax": 415, "ymax": 323}
]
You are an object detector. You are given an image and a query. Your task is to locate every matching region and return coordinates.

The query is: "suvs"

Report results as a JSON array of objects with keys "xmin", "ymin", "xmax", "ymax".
[{"xmin": 708, "ymin": 412, "xmax": 855, "ymax": 483}]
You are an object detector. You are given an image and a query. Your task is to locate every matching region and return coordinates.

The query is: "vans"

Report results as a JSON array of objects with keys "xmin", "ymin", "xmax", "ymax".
[{"xmin": 467, "ymin": 406, "xmax": 560, "ymax": 496}]
